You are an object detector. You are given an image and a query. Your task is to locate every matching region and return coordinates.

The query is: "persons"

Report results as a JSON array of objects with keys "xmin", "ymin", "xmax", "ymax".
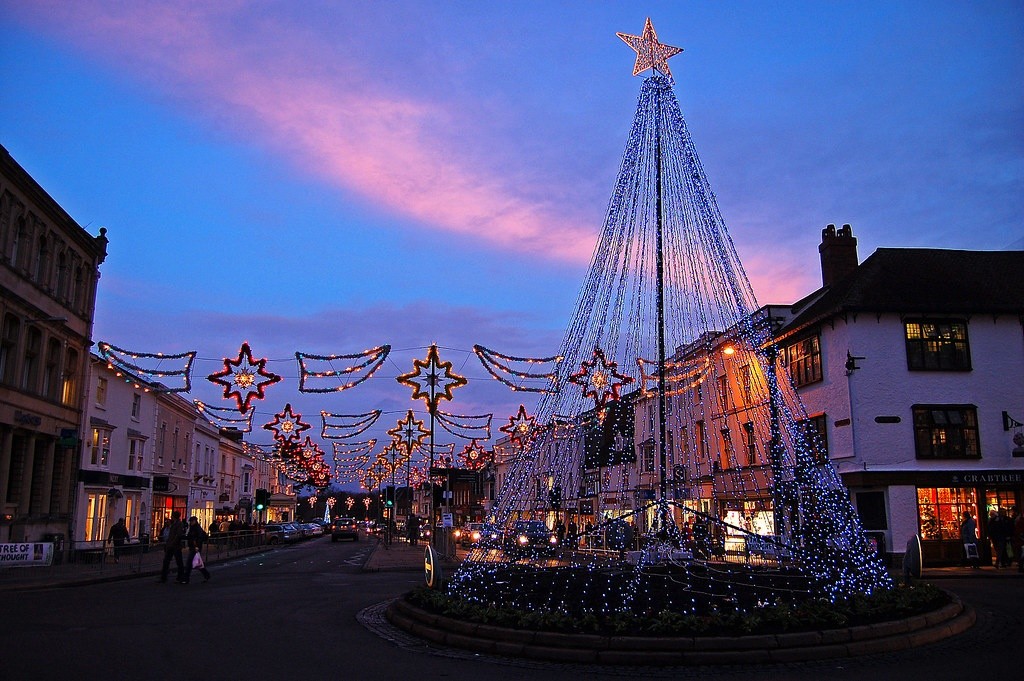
[
  {"xmin": 108, "ymin": 517, "xmax": 130, "ymax": 564},
  {"xmin": 151, "ymin": 510, "xmax": 281, "ymax": 586},
  {"xmin": 392, "ymin": 523, "xmax": 401, "ymax": 535},
  {"xmin": 408, "ymin": 514, "xmax": 418, "ymax": 546},
  {"xmin": 960, "ymin": 506, "xmax": 1024, "ymax": 565},
  {"xmin": 554, "ymin": 514, "xmax": 726, "ymax": 561}
]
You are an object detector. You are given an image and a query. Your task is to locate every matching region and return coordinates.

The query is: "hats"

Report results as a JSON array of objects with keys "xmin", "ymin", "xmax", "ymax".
[
  {"xmin": 189, "ymin": 516, "xmax": 198, "ymax": 522},
  {"xmin": 172, "ymin": 511, "xmax": 181, "ymax": 520}
]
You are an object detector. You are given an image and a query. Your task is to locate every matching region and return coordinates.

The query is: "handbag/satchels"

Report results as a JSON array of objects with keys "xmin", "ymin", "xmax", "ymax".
[
  {"xmin": 198, "ymin": 525, "xmax": 209, "ymax": 543},
  {"xmin": 191, "ymin": 551, "xmax": 205, "ymax": 571},
  {"xmin": 1005, "ymin": 540, "xmax": 1015, "ymax": 558},
  {"xmin": 1020, "ymin": 541, "xmax": 1024, "ymax": 559},
  {"xmin": 964, "ymin": 542, "xmax": 979, "ymax": 559}
]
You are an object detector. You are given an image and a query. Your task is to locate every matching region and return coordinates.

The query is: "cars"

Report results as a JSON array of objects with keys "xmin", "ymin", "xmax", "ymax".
[
  {"xmin": 401, "ymin": 515, "xmax": 560, "ymax": 556},
  {"xmin": 330, "ymin": 518, "xmax": 359, "ymax": 542},
  {"xmin": 248, "ymin": 518, "xmax": 326, "ymax": 547}
]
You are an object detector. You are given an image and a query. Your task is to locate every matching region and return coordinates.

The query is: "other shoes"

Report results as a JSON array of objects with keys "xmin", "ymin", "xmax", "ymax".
[
  {"xmin": 203, "ymin": 576, "xmax": 211, "ymax": 583},
  {"xmin": 152, "ymin": 579, "xmax": 166, "ymax": 585},
  {"xmin": 113, "ymin": 558, "xmax": 119, "ymax": 564},
  {"xmin": 175, "ymin": 576, "xmax": 185, "ymax": 582},
  {"xmin": 178, "ymin": 579, "xmax": 188, "ymax": 585}
]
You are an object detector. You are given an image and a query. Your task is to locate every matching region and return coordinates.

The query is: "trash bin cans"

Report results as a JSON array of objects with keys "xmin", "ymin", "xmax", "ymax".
[{"xmin": 42, "ymin": 533, "xmax": 64, "ymax": 565}]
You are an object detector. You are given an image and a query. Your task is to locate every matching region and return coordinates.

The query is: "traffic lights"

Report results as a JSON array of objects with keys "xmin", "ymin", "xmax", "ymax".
[
  {"xmin": 265, "ymin": 488, "xmax": 271, "ymax": 509},
  {"xmin": 385, "ymin": 486, "xmax": 395, "ymax": 508},
  {"xmin": 255, "ymin": 488, "xmax": 266, "ymax": 511}
]
